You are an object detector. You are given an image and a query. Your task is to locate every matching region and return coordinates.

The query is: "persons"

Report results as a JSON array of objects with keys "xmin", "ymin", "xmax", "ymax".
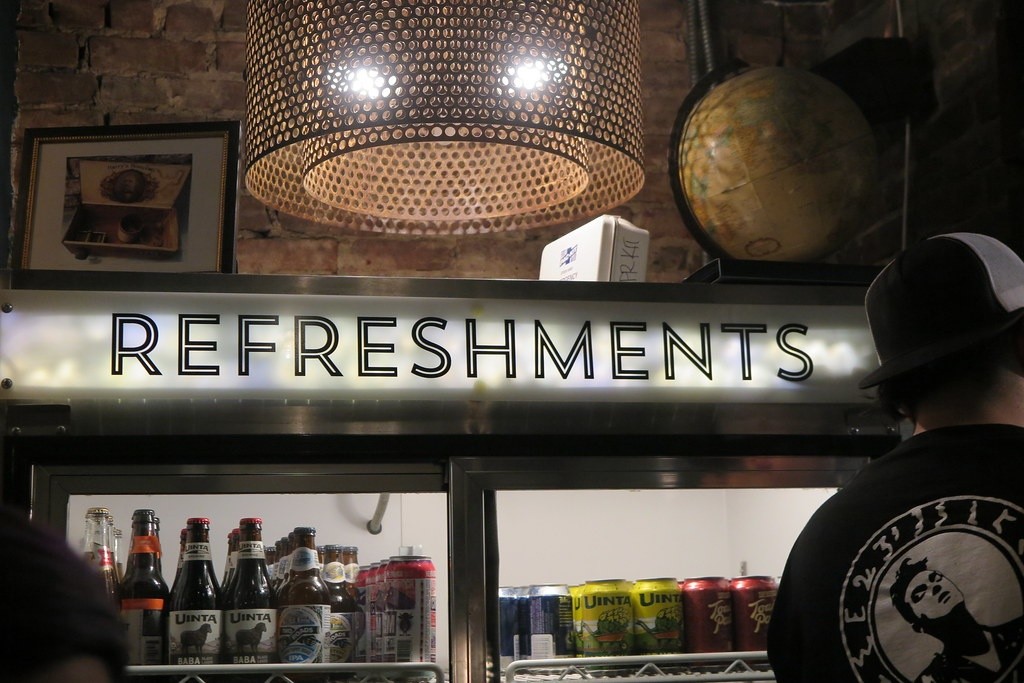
[
  {"xmin": 0, "ymin": 516, "xmax": 127, "ymax": 683},
  {"xmin": 766, "ymin": 233, "xmax": 1024, "ymax": 682}
]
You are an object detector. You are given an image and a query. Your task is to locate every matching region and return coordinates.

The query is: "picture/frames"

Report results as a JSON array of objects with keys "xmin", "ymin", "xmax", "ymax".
[{"xmin": 11, "ymin": 120, "xmax": 240, "ymax": 274}]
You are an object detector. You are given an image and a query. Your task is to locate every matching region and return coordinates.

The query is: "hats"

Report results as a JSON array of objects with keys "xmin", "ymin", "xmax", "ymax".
[{"xmin": 856, "ymin": 228, "xmax": 1024, "ymax": 392}]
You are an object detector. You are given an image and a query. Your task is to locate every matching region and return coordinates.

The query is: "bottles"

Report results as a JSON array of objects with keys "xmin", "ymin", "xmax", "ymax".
[{"xmin": 76, "ymin": 504, "xmax": 366, "ymax": 678}]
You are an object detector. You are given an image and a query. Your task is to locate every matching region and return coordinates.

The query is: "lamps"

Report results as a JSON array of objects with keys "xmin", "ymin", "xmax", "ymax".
[{"xmin": 242, "ymin": 0, "xmax": 646, "ymax": 241}]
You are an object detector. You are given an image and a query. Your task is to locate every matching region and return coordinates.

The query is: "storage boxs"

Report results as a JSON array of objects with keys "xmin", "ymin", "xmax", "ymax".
[{"xmin": 538, "ymin": 214, "xmax": 649, "ymax": 281}]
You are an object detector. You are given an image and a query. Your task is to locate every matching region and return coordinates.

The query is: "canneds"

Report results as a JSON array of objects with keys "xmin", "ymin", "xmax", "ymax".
[
  {"xmin": 353, "ymin": 555, "xmax": 436, "ymax": 683},
  {"xmin": 501, "ymin": 575, "xmax": 777, "ymax": 676}
]
